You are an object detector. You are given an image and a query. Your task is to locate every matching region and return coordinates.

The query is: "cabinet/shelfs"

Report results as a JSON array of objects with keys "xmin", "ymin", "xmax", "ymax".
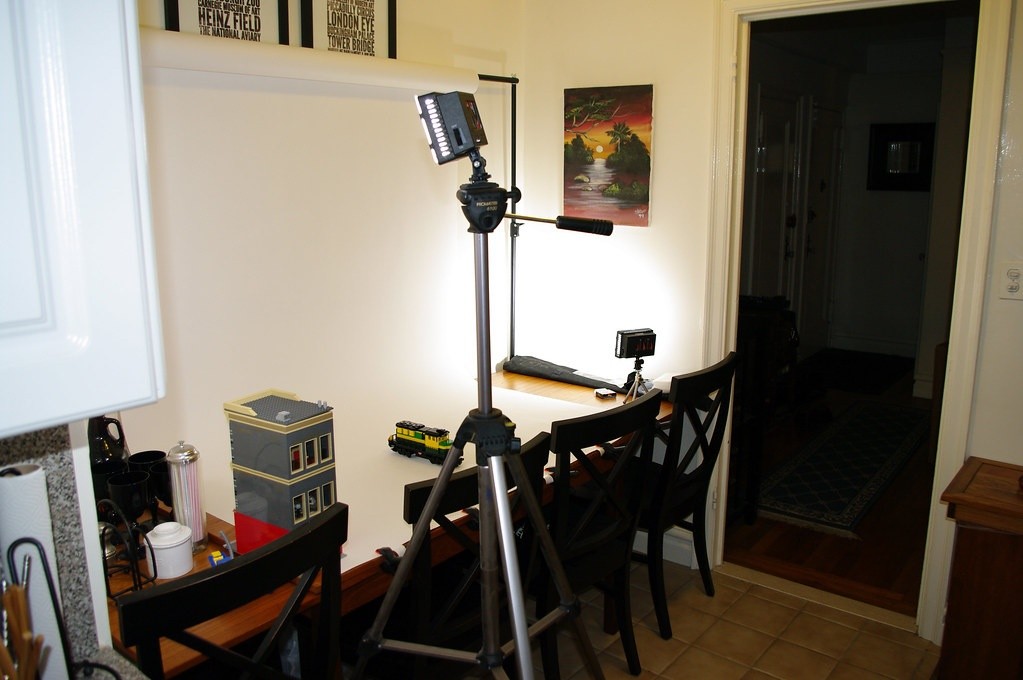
[
  {"xmin": 1, "ymin": 0, "xmax": 166, "ymax": 441},
  {"xmin": 936, "ymin": 456, "xmax": 1023, "ymax": 680}
]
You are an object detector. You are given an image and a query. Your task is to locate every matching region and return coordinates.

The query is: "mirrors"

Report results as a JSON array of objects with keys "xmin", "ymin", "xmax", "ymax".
[{"xmin": 864, "ymin": 121, "xmax": 936, "ymax": 192}]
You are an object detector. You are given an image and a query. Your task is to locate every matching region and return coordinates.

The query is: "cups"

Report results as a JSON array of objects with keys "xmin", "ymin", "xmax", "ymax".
[{"xmin": 108, "ymin": 450, "xmax": 171, "ymax": 521}]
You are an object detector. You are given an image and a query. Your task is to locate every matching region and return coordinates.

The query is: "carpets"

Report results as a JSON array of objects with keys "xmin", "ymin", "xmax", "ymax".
[{"xmin": 753, "ymin": 399, "xmax": 931, "ymax": 539}]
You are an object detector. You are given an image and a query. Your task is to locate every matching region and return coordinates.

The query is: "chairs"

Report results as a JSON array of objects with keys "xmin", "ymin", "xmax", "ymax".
[{"xmin": 114, "ymin": 349, "xmax": 737, "ymax": 680}]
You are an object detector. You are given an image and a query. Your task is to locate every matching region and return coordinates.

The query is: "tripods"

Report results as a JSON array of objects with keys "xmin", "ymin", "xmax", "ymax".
[
  {"xmin": 623, "ymin": 359, "xmax": 649, "ymax": 404},
  {"xmin": 350, "ymin": 182, "xmax": 613, "ymax": 680}
]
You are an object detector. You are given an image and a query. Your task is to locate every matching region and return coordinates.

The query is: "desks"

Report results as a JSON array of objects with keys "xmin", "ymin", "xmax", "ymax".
[{"xmin": 106, "ymin": 367, "xmax": 673, "ymax": 680}]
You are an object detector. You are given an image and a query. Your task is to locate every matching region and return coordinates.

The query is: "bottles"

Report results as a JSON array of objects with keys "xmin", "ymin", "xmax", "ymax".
[
  {"xmin": 88, "ymin": 415, "xmax": 131, "ymax": 523},
  {"xmin": 167, "ymin": 440, "xmax": 209, "ymax": 554}
]
